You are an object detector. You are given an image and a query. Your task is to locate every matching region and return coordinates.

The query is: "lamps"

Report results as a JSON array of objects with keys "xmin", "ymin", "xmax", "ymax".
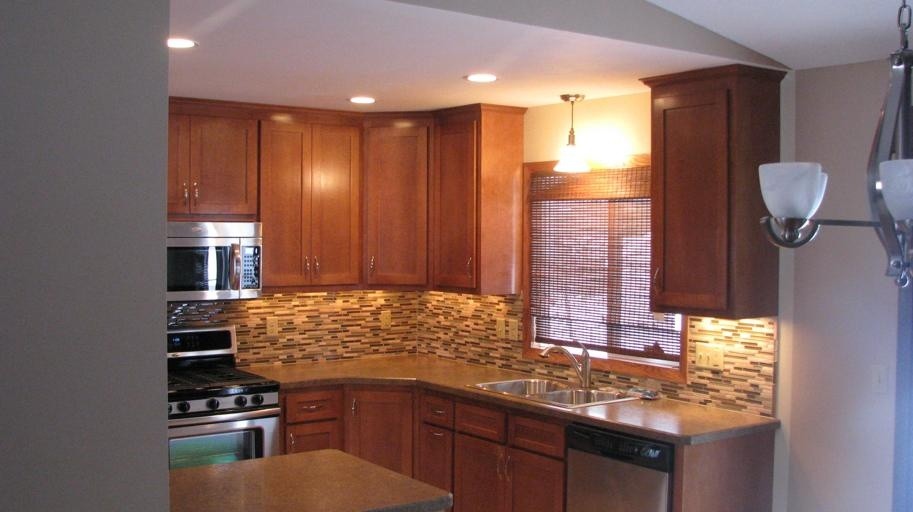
[
  {"xmin": 552, "ymin": 91, "xmax": 588, "ymax": 178},
  {"xmin": 752, "ymin": 0, "xmax": 910, "ymax": 287}
]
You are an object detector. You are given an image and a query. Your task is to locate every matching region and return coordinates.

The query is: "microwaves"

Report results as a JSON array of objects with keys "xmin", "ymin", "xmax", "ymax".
[{"xmin": 166, "ymin": 221, "xmax": 264, "ymax": 299}]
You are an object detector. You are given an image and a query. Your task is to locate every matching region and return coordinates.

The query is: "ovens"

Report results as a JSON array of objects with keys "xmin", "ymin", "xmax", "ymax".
[{"xmin": 168, "ymin": 406, "xmax": 282, "ymax": 472}]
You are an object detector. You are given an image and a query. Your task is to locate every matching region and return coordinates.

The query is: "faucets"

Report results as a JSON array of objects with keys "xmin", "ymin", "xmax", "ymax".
[{"xmin": 538, "ymin": 340, "xmax": 590, "ymax": 387}]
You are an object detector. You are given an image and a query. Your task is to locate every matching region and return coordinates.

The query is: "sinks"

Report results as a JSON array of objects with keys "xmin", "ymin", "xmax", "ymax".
[
  {"xmin": 532, "ymin": 391, "xmax": 640, "ymax": 408},
  {"xmin": 463, "ymin": 378, "xmax": 569, "ymax": 395}
]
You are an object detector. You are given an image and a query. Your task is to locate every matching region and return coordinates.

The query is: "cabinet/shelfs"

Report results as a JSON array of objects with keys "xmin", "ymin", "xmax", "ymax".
[
  {"xmin": 346, "ymin": 382, "xmax": 420, "ymax": 477},
  {"xmin": 259, "ymin": 108, "xmax": 361, "ymax": 294},
  {"xmin": 411, "ymin": 389, "xmax": 455, "ymax": 487},
  {"xmin": 430, "ymin": 103, "xmax": 527, "ymax": 301},
  {"xmin": 363, "ymin": 106, "xmax": 427, "ymax": 292},
  {"xmin": 283, "ymin": 385, "xmax": 345, "ymax": 455},
  {"xmin": 639, "ymin": 58, "xmax": 779, "ymax": 319},
  {"xmin": 166, "ymin": 96, "xmax": 256, "ymax": 219},
  {"xmin": 453, "ymin": 400, "xmax": 573, "ymax": 510}
]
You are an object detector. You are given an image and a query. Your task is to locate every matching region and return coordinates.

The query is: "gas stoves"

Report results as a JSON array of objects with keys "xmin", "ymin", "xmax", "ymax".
[{"xmin": 168, "ymin": 361, "xmax": 280, "ymax": 416}]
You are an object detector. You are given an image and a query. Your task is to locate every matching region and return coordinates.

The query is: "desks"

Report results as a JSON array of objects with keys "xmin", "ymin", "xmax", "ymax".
[{"xmin": 170, "ymin": 447, "xmax": 454, "ymax": 512}]
